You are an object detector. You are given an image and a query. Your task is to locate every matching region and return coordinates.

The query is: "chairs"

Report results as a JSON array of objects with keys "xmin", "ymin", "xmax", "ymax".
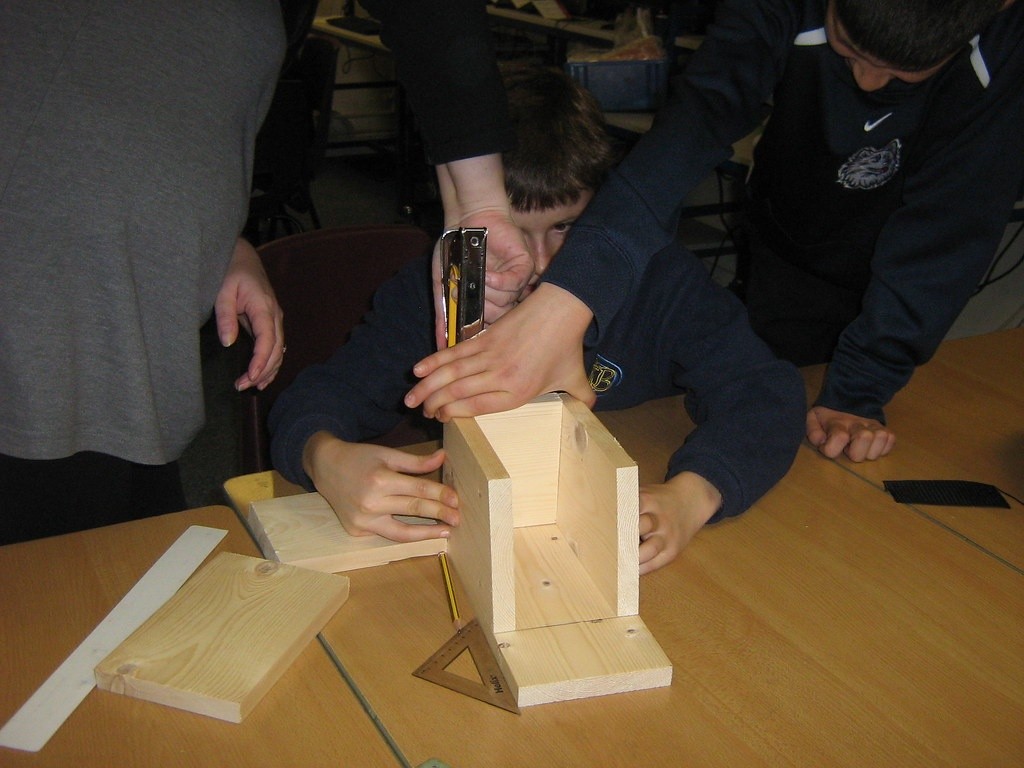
[
  {"xmin": 242, "ymin": 0, "xmax": 337, "ymax": 243},
  {"xmin": 244, "ymin": 223, "xmax": 444, "ymax": 474}
]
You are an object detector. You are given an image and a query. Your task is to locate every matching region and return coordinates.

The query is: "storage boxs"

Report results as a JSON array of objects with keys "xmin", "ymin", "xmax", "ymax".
[{"xmin": 565, "ymin": 60, "xmax": 673, "ymax": 111}]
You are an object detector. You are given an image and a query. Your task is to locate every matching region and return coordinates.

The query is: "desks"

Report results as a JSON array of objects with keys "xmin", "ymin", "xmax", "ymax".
[
  {"xmin": 312, "ymin": 4, "xmax": 708, "ymax": 219},
  {"xmin": 0, "ymin": 321, "xmax": 1024, "ymax": 768},
  {"xmin": 602, "ymin": 108, "xmax": 771, "ymax": 297}
]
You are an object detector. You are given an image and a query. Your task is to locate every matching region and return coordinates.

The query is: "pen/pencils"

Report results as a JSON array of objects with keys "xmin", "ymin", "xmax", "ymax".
[{"xmin": 437, "ymin": 551, "xmax": 463, "ymax": 634}]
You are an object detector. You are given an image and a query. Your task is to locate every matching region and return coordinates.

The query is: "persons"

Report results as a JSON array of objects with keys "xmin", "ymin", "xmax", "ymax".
[{"xmin": 0, "ymin": 1, "xmax": 1024, "ymax": 575}]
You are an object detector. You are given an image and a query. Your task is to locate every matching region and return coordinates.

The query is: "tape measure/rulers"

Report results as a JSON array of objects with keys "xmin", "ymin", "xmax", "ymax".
[{"xmin": 411, "ymin": 618, "xmax": 522, "ymax": 715}]
